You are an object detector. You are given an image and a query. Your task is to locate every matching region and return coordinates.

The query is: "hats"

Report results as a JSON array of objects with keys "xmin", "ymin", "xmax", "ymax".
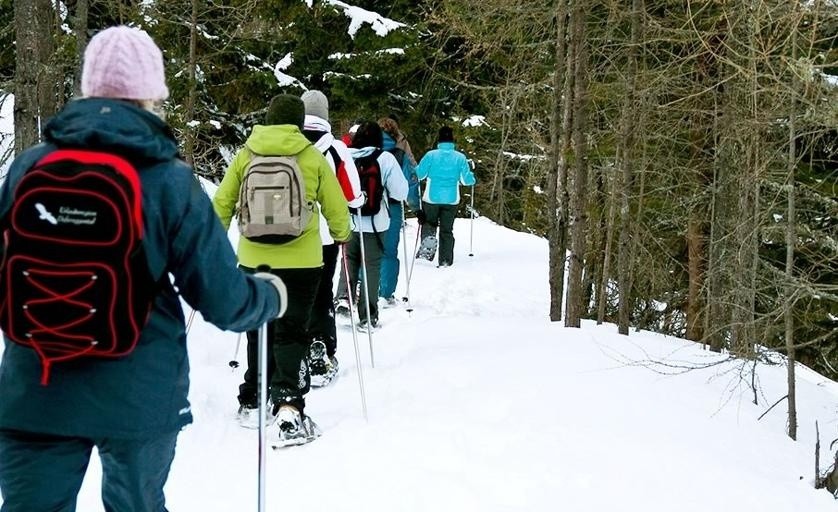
[
  {"xmin": 80, "ymin": 25, "xmax": 169, "ymax": 104},
  {"xmin": 438, "ymin": 126, "xmax": 454, "ymax": 143},
  {"xmin": 265, "ymin": 94, "xmax": 307, "ymax": 128},
  {"xmin": 300, "ymin": 89, "xmax": 329, "ymax": 123}
]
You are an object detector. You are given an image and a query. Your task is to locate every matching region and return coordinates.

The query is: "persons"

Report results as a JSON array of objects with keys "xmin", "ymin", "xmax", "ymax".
[
  {"xmin": 340, "ymin": 110, "xmax": 475, "ymax": 330},
  {"xmin": 0, "ymin": 20, "xmax": 289, "ymax": 512},
  {"xmin": 294, "ymin": 85, "xmax": 367, "ymax": 389},
  {"xmin": 212, "ymin": 92, "xmax": 353, "ymax": 449}
]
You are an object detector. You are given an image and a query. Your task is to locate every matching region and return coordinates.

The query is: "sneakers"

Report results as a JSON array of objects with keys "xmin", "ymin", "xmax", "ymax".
[
  {"xmin": 378, "ymin": 295, "xmax": 407, "ymax": 312},
  {"xmin": 306, "ymin": 342, "xmax": 332, "ymax": 376},
  {"xmin": 276, "ymin": 405, "xmax": 306, "ymax": 439},
  {"xmin": 238, "ymin": 392, "xmax": 265, "ymax": 418},
  {"xmin": 356, "ymin": 316, "xmax": 379, "ymax": 330},
  {"xmin": 335, "ymin": 295, "xmax": 350, "ymax": 312}
]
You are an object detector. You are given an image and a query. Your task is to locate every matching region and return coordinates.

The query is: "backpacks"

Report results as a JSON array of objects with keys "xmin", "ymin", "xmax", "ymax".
[
  {"xmin": 234, "ymin": 154, "xmax": 309, "ymax": 245},
  {"xmin": 347, "ymin": 148, "xmax": 385, "ymax": 218},
  {"xmin": 0, "ymin": 148, "xmax": 152, "ymax": 389}
]
